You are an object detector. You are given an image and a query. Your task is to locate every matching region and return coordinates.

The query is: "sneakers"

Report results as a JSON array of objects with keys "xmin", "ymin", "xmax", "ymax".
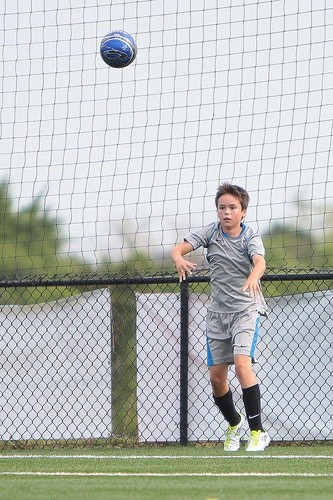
[
  {"xmin": 224, "ymin": 412, "xmax": 247, "ymax": 451},
  {"xmin": 245, "ymin": 429, "xmax": 271, "ymax": 451}
]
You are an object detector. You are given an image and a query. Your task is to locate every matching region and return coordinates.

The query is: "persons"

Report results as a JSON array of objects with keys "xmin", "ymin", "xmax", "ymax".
[{"xmin": 171, "ymin": 182, "xmax": 271, "ymax": 451}]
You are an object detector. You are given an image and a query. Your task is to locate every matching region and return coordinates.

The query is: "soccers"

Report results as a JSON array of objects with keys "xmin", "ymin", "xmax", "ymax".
[{"xmin": 100, "ymin": 29, "xmax": 137, "ymax": 68}]
